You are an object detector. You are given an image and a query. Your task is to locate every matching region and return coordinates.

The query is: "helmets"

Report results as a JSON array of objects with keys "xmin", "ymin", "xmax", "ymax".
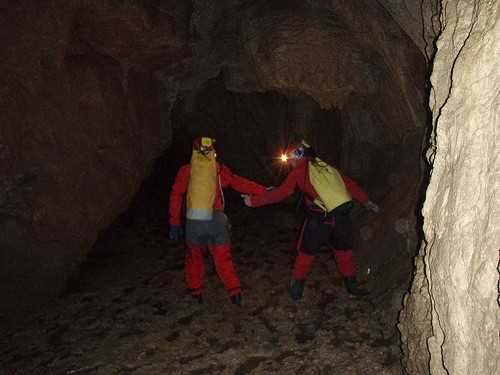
[
  {"xmin": 192, "ymin": 135, "xmax": 215, "ymax": 151},
  {"xmin": 284, "ymin": 142, "xmax": 306, "ymax": 165}
]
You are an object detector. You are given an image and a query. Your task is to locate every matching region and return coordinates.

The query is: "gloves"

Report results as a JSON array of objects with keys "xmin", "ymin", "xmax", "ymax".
[
  {"xmin": 364, "ymin": 201, "xmax": 380, "ymax": 213},
  {"xmin": 267, "ymin": 187, "xmax": 275, "ymax": 192},
  {"xmin": 169, "ymin": 225, "xmax": 182, "ymax": 241}
]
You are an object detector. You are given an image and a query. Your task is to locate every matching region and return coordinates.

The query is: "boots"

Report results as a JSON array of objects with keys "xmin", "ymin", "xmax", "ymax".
[
  {"xmin": 289, "ymin": 277, "xmax": 306, "ymax": 301},
  {"xmin": 343, "ymin": 275, "xmax": 358, "ymax": 296}
]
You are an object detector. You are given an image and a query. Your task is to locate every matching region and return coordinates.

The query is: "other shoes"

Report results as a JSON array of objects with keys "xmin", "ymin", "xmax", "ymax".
[
  {"xmin": 231, "ymin": 293, "xmax": 242, "ymax": 306},
  {"xmin": 194, "ymin": 293, "xmax": 204, "ymax": 305}
]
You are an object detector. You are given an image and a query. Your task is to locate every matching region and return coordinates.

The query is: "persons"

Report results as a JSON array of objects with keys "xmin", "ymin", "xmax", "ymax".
[
  {"xmin": 241, "ymin": 141, "xmax": 382, "ymax": 303},
  {"xmin": 169, "ymin": 138, "xmax": 276, "ymax": 307}
]
